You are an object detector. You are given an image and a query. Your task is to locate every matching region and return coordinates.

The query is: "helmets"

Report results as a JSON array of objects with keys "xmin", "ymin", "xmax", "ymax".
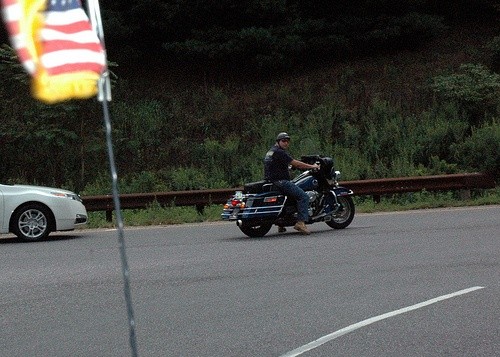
[{"xmin": 277, "ymin": 132, "xmax": 290, "ymax": 139}]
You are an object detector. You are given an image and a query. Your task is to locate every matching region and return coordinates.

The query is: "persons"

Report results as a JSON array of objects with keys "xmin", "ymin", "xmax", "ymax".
[{"xmin": 264, "ymin": 132, "xmax": 321, "ymax": 235}]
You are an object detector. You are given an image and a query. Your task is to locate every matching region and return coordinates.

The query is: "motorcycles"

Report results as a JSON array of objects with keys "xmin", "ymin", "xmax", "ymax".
[{"xmin": 221, "ymin": 154, "xmax": 356, "ymax": 238}]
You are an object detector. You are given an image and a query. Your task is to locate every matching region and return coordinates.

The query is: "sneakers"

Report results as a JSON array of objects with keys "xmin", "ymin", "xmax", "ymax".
[
  {"xmin": 277, "ymin": 227, "xmax": 286, "ymax": 233},
  {"xmin": 294, "ymin": 221, "xmax": 311, "ymax": 235}
]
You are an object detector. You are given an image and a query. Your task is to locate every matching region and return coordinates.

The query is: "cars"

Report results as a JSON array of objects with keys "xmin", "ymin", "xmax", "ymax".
[{"xmin": 0, "ymin": 184, "xmax": 89, "ymax": 241}]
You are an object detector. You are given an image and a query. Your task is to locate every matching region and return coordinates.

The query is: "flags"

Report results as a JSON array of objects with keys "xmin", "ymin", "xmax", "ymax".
[{"xmin": 0, "ymin": 0, "xmax": 108, "ymax": 104}]
